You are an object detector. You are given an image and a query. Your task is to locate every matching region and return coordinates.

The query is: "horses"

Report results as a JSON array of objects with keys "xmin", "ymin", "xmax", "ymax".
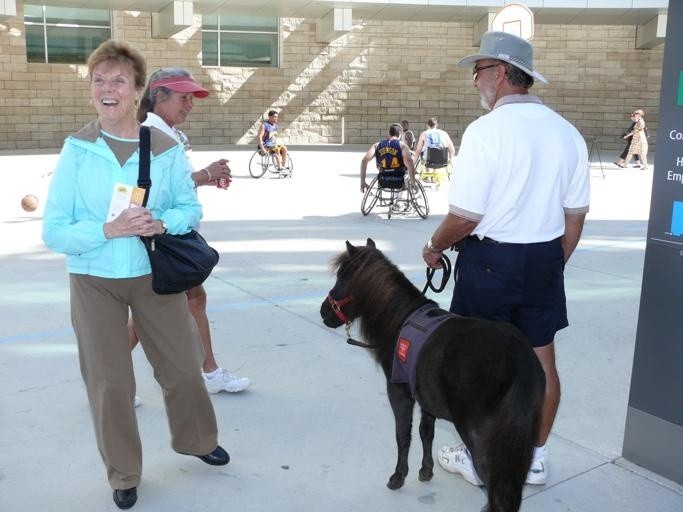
[{"xmin": 319, "ymin": 237, "xmax": 548, "ymax": 512}]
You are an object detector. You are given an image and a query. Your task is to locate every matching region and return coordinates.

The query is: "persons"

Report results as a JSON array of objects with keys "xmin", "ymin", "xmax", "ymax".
[
  {"xmin": 258, "ymin": 110, "xmax": 290, "ymax": 170},
  {"xmin": 126, "ymin": 67, "xmax": 251, "ymax": 408},
  {"xmin": 618, "ymin": 110, "xmax": 649, "ymax": 170},
  {"xmin": 400, "ymin": 120, "xmax": 415, "ymax": 155},
  {"xmin": 413, "ymin": 118, "xmax": 455, "ymax": 183},
  {"xmin": 614, "ymin": 112, "xmax": 640, "ymax": 167},
  {"xmin": 361, "ymin": 123, "xmax": 418, "ymax": 193},
  {"xmin": 422, "ymin": 30, "xmax": 590, "ymax": 487},
  {"xmin": 42, "ymin": 38, "xmax": 230, "ymax": 509}
]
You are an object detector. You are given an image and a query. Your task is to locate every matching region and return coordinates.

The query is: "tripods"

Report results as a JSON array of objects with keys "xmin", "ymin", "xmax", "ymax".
[{"xmin": 588, "ymin": 137, "xmax": 605, "ymax": 180}]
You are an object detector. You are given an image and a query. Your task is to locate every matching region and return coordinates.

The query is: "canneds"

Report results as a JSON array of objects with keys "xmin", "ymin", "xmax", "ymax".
[{"xmin": 217, "ymin": 177, "xmax": 228, "ymax": 189}]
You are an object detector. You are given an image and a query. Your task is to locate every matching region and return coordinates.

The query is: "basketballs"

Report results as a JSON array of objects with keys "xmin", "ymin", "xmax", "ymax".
[{"xmin": 22, "ymin": 194, "xmax": 38, "ymax": 211}]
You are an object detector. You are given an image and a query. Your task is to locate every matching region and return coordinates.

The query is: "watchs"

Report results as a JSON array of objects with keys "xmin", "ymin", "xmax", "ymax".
[
  {"xmin": 157, "ymin": 219, "xmax": 168, "ymax": 235},
  {"xmin": 428, "ymin": 239, "xmax": 442, "ymax": 253}
]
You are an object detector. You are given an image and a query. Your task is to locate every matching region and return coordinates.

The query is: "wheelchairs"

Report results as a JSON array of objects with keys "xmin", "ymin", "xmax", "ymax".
[
  {"xmin": 248, "ymin": 133, "xmax": 292, "ymax": 179},
  {"xmin": 360, "ymin": 130, "xmax": 454, "ymax": 221}
]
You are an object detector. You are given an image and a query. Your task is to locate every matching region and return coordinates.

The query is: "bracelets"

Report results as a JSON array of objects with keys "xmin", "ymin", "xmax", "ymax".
[{"xmin": 201, "ymin": 168, "xmax": 211, "ymax": 182}]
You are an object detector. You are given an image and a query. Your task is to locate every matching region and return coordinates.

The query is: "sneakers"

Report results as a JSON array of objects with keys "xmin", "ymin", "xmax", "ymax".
[
  {"xmin": 203, "ymin": 369, "xmax": 250, "ymax": 393},
  {"xmin": 438, "ymin": 443, "xmax": 486, "ymax": 486},
  {"xmin": 524, "ymin": 458, "xmax": 547, "ymax": 485}
]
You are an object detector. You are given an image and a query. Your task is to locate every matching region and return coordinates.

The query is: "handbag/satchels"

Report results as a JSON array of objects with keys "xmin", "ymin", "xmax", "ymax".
[{"xmin": 138, "ymin": 227, "xmax": 219, "ymax": 294}]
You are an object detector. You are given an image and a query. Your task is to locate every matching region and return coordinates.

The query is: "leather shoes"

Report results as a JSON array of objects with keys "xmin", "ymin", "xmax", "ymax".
[
  {"xmin": 615, "ymin": 162, "xmax": 627, "ymax": 168},
  {"xmin": 173, "ymin": 446, "xmax": 229, "ymax": 466},
  {"xmin": 113, "ymin": 487, "xmax": 137, "ymax": 509},
  {"xmin": 633, "ymin": 166, "xmax": 648, "ymax": 169}
]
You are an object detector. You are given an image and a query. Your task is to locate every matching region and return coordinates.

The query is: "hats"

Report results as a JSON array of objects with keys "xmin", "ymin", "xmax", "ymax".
[
  {"xmin": 459, "ymin": 32, "xmax": 549, "ymax": 84},
  {"xmin": 150, "ymin": 77, "xmax": 208, "ymax": 98}
]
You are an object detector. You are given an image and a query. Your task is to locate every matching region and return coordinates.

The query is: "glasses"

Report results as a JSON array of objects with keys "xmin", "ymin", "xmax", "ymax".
[{"xmin": 474, "ymin": 64, "xmax": 497, "ymax": 80}]
